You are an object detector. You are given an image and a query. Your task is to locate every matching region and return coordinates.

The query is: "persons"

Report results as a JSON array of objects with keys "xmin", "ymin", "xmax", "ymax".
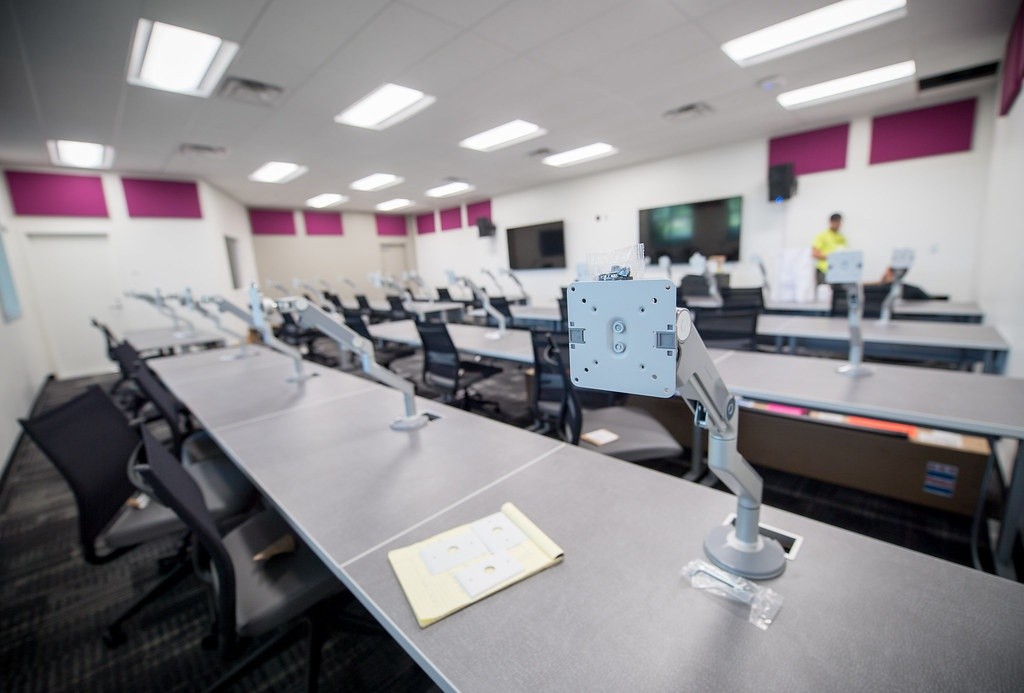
[{"xmin": 811, "ymin": 213, "xmax": 848, "ymax": 284}]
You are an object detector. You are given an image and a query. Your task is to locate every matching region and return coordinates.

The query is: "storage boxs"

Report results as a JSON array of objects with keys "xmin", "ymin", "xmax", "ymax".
[{"xmin": 627, "ymin": 393, "xmax": 992, "ymax": 515}]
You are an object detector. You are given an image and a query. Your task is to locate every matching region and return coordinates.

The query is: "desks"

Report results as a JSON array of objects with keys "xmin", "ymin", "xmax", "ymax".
[
  {"xmin": 212, "ymin": 385, "xmax": 567, "ymax": 576},
  {"xmin": 681, "ymin": 292, "xmax": 831, "ymax": 316},
  {"xmin": 146, "ymin": 343, "xmax": 379, "ymax": 435},
  {"xmin": 754, "ymin": 313, "xmax": 1011, "ymax": 375},
  {"xmin": 468, "ymin": 305, "xmax": 527, "ymax": 327},
  {"xmin": 337, "ymin": 442, "xmax": 1024, "ymax": 693},
  {"xmin": 368, "ymin": 319, "xmax": 466, "ymax": 401},
  {"xmin": 513, "ymin": 306, "xmax": 561, "ymax": 331},
  {"xmin": 420, "ymin": 325, "xmax": 540, "ymax": 431},
  {"xmin": 891, "ymin": 299, "xmax": 985, "ymax": 323},
  {"xmin": 698, "ymin": 351, "xmax": 1024, "ymax": 581},
  {"xmin": 92, "ymin": 308, "xmax": 231, "ymax": 426}
]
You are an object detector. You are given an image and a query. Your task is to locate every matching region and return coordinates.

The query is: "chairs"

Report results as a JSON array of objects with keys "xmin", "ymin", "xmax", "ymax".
[
  {"xmin": 525, "ymin": 327, "xmax": 629, "ymax": 425},
  {"xmin": 113, "ymin": 339, "xmax": 148, "ymax": 420},
  {"xmin": 125, "ymin": 422, "xmax": 386, "ymax": 693},
  {"xmin": 486, "ymin": 296, "xmax": 531, "ymax": 371},
  {"xmin": 544, "ymin": 333, "xmax": 683, "ymax": 474},
  {"xmin": 301, "ymin": 288, "xmax": 486, "ymax": 325},
  {"xmin": 694, "ymin": 305, "xmax": 760, "ymax": 352},
  {"xmin": 680, "ymin": 273, "xmax": 731, "ymax": 297},
  {"xmin": 17, "ymin": 382, "xmax": 266, "ymax": 655},
  {"xmin": 719, "ymin": 287, "xmax": 769, "ymax": 314},
  {"xmin": 344, "ymin": 317, "xmax": 419, "ymax": 394},
  {"xmin": 276, "ymin": 305, "xmax": 339, "ymax": 367},
  {"xmin": 412, "ymin": 316, "xmax": 504, "ymax": 414},
  {"xmin": 124, "ymin": 357, "xmax": 206, "ymax": 466}
]
